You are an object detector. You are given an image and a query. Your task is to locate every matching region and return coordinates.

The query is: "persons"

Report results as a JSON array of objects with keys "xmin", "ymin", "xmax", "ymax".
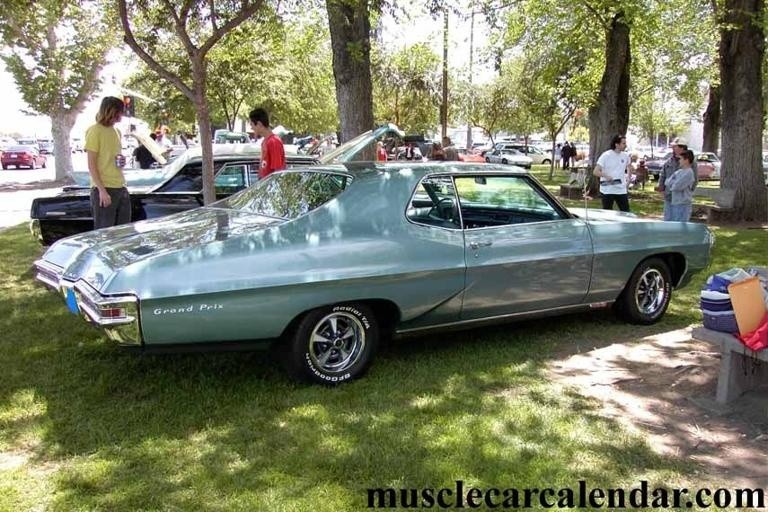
[
  {"xmin": 632, "ymin": 159, "xmax": 650, "ymax": 192},
  {"xmin": 665, "ymin": 150, "xmax": 696, "ymax": 222},
  {"xmin": 82, "ymin": 95, "xmax": 133, "ymax": 231},
  {"xmin": 553, "ymin": 141, "xmax": 578, "ymax": 171},
  {"xmin": 248, "ymin": 107, "xmax": 287, "ymax": 182},
  {"xmin": 120, "ymin": 126, "xmax": 201, "ymax": 170},
  {"xmin": 293, "ymin": 133, "xmax": 485, "ymax": 162},
  {"xmin": 592, "ymin": 133, "xmax": 631, "ymax": 213},
  {"xmin": 654, "ymin": 137, "xmax": 698, "ymax": 222}
]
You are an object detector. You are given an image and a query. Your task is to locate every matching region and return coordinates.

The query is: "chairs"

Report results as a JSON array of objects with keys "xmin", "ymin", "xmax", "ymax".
[{"xmin": 693, "ymin": 186, "xmax": 739, "ymax": 224}]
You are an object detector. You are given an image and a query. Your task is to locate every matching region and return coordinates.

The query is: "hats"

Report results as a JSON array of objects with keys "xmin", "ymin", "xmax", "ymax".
[{"xmin": 669, "ymin": 137, "xmax": 690, "ymax": 147}]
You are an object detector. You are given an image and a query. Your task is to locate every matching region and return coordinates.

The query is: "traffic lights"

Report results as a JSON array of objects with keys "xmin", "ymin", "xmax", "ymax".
[{"xmin": 122, "ymin": 94, "xmax": 135, "ymax": 117}]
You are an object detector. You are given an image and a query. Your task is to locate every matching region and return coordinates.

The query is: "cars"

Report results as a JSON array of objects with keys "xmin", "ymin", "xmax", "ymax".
[
  {"xmin": 33, "ymin": 159, "xmax": 715, "ymax": 387},
  {"xmin": 0, "ymin": 145, "xmax": 47, "ymax": 169},
  {"xmin": 30, "ymin": 123, "xmax": 407, "ymax": 246},
  {"xmin": 644, "ymin": 150, "xmax": 722, "ymax": 182},
  {"xmin": 470, "ymin": 138, "xmax": 553, "ymax": 169}
]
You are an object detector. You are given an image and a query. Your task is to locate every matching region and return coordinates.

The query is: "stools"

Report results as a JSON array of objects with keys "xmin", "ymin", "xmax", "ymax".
[
  {"xmin": 692, "ymin": 326, "xmax": 766, "ymax": 404},
  {"xmin": 557, "ymin": 181, "xmax": 583, "ymax": 200}
]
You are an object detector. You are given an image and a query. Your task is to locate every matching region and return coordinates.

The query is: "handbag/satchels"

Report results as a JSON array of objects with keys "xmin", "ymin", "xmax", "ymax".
[{"xmin": 701, "ymin": 268, "xmax": 768, "ymax": 351}]
